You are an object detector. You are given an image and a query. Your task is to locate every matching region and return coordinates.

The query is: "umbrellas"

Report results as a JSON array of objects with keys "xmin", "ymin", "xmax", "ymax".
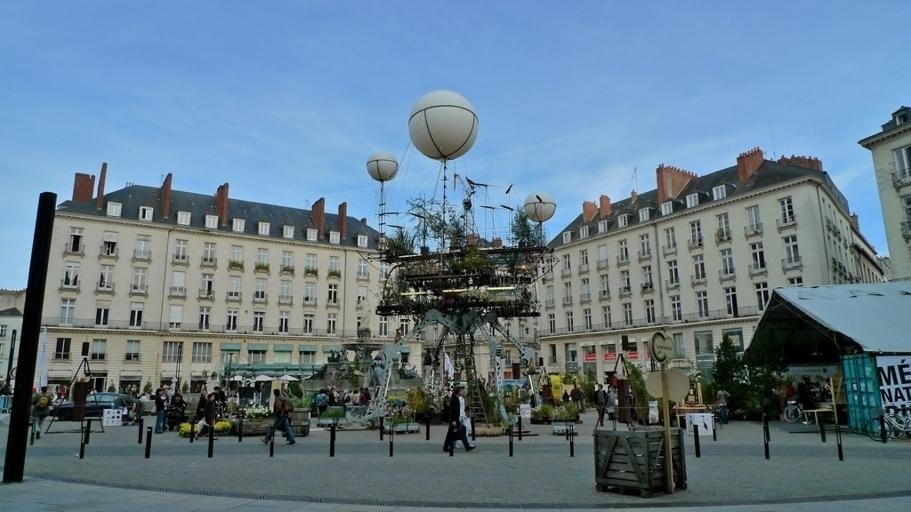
[{"xmin": 227, "ymin": 374, "xmax": 299, "ymax": 382}]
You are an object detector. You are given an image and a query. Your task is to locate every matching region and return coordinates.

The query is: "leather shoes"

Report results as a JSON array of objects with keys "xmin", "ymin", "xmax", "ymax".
[
  {"xmin": 443, "ymin": 445, "xmax": 460, "ymax": 452},
  {"xmin": 465, "ymin": 444, "xmax": 476, "ymax": 451}
]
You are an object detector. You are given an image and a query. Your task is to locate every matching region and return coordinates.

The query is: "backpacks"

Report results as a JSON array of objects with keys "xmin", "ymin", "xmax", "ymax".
[
  {"xmin": 36, "ymin": 394, "xmax": 49, "ymax": 408},
  {"xmin": 276, "ymin": 398, "xmax": 294, "ymax": 412}
]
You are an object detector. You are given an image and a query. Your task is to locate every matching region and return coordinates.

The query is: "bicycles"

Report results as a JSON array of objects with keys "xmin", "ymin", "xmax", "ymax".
[
  {"xmin": 866, "ymin": 407, "xmax": 911, "ymax": 441},
  {"xmin": 783, "ymin": 401, "xmax": 803, "ymax": 422}
]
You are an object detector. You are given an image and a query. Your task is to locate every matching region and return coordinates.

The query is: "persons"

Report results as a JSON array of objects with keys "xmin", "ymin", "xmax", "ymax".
[
  {"xmin": 197, "ymin": 386, "xmax": 225, "ymax": 440},
  {"xmin": 155, "ymin": 383, "xmax": 187, "ymax": 434},
  {"xmin": 443, "ymin": 386, "xmax": 476, "ymax": 452},
  {"xmin": 716, "ymin": 390, "xmax": 731, "ymax": 424},
  {"xmin": 55, "ymin": 383, "xmax": 70, "ymax": 402},
  {"xmin": 316, "ymin": 387, "xmax": 370, "ymax": 413},
  {"xmin": 562, "ymin": 384, "xmax": 585, "ymax": 409},
  {"xmin": 786, "ymin": 375, "xmax": 831, "ymax": 410},
  {"xmin": 119, "ymin": 384, "xmax": 137, "ymax": 399},
  {"xmin": 258, "ymin": 389, "xmax": 296, "ymax": 445},
  {"xmin": 594, "ymin": 384, "xmax": 616, "ymax": 426},
  {"xmin": 0, "ymin": 385, "xmax": 12, "ymax": 413},
  {"xmin": 30, "ymin": 386, "xmax": 52, "ymax": 445}
]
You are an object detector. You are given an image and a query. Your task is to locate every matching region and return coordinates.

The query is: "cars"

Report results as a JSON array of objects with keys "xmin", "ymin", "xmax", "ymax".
[{"xmin": 48, "ymin": 392, "xmax": 143, "ymax": 421}]
